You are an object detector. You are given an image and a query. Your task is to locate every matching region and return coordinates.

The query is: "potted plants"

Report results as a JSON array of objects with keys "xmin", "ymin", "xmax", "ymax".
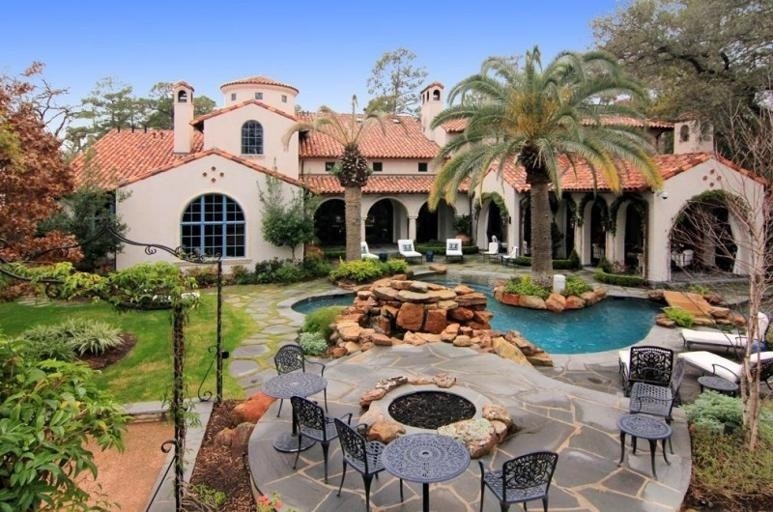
[{"xmin": 451, "ymin": 213, "xmax": 471, "ymax": 246}]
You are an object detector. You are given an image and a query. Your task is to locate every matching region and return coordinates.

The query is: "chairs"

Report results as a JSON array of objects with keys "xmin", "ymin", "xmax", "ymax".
[
  {"xmin": 617, "ymin": 308, "xmax": 773, "ymax": 457},
  {"xmin": 360, "ymin": 235, "xmax": 521, "ymax": 269},
  {"xmin": 592, "ymin": 240, "xmax": 605, "ymax": 260},
  {"xmin": 670, "ymin": 248, "xmax": 697, "ymax": 280}
]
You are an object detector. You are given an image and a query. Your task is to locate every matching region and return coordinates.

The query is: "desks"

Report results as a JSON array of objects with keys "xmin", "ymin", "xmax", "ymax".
[
  {"xmin": 617, "ymin": 412, "xmax": 673, "ymax": 483},
  {"xmin": 262, "ymin": 372, "xmax": 327, "ymax": 454}
]
